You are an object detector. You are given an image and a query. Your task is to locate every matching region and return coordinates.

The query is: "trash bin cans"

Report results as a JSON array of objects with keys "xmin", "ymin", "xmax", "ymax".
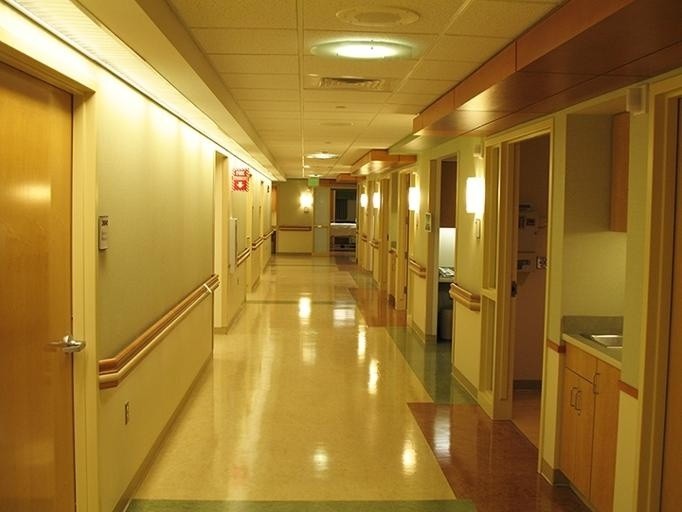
[{"xmin": 440, "ymin": 307, "xmax": 453, "ymax": 340}]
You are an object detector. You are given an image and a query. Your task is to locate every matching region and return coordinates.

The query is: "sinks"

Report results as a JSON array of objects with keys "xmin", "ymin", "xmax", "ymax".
[{"xmin": 590, "ymin": 334, "xmax": 623, "ymax": 349}]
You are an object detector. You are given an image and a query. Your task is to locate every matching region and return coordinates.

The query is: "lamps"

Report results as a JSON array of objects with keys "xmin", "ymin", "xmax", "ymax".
[
  {"xmin": 466, "ymin": 177, "xmax": 485, "ymax": 213},
  {"xmin": 361, "ymin": 194, "xmax": 368, "ymax": 207},
  {"xmin": 408, "ymin": 187, "xmax": 420, "ymax": 211},
  {"xmin": 373, "ymin": 192, "xmax": 380, "ymax": 208}
]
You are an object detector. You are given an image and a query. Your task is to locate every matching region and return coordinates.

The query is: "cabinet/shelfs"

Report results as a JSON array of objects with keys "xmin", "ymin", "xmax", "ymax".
[{"xmin": 559, "ymin": 342, "xmax": 620, "ymax": 512}]
